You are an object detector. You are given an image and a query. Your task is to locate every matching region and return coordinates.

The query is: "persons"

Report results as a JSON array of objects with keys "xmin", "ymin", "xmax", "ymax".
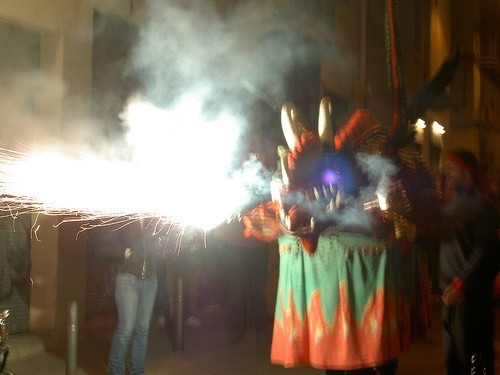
[
  {"xmin": 405, "ymin": 171, "xmax": 448, "ymax": 305},
  {"xmin": 422, "ymin": 147, "xmax": 500, "ymax": 375},
  {"xmin": 102, "ymin": 224, "xmax": 160, "ymax": 373}
]
